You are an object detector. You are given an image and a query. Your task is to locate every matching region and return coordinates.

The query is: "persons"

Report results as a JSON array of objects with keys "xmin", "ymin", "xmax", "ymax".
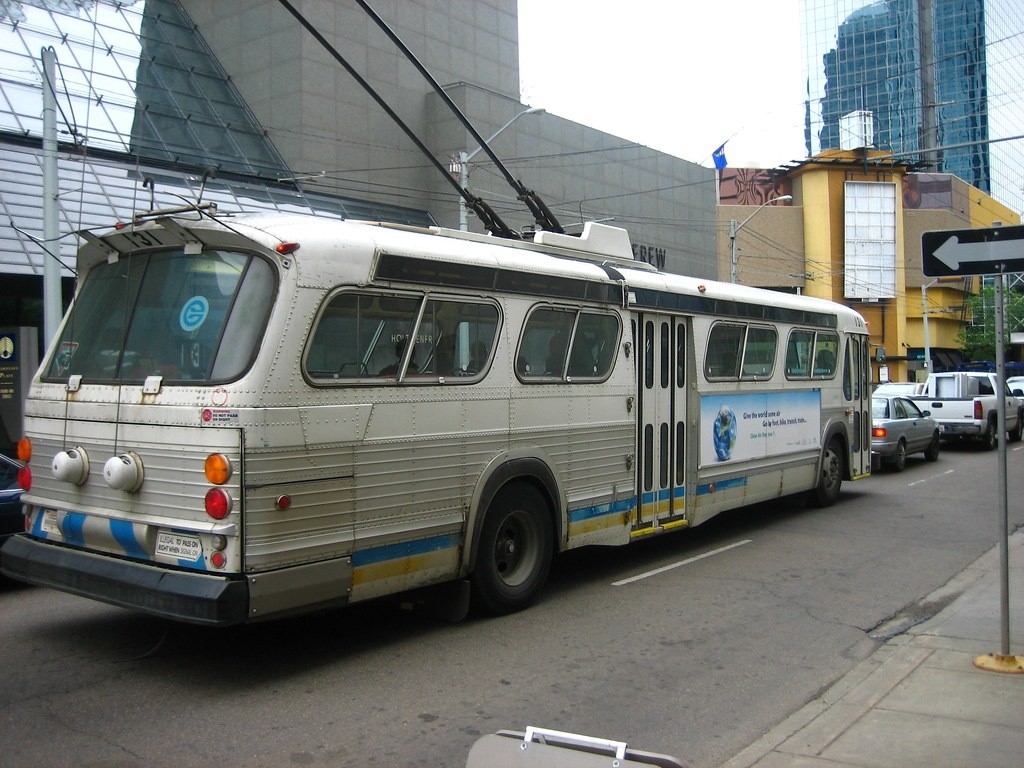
[
  {"xmin": 435, "ymin": 335, "xmax": 488, "ymax": 376},
  {"xmin": 518, "ymin": 356, "xmax": 530, "ymax": 376},
  {"xmin": 703, "ymin": 343, "xmax": 836, "ymax": 377},
  {"xmin": 379, "ymin": 339, "xmax": 420, "ymax": 376}
]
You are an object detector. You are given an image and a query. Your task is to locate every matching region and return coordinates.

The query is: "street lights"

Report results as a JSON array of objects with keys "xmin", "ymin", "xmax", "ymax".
[
  {"xmin": 458, "ymin": 107, "xmax": 548, "ymax": 235},
  {"xmin": 727, "ymin": 196, "xmax": 794, "ymax": 287}
]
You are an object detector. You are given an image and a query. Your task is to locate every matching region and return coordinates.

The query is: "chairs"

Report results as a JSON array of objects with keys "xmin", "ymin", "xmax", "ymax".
[
  {"xmin": 379, "ymin": 340, "xmax": 417, "ymax": 372},
  {"xmin": 549, "ymin": 328, "xmax": 596, "ymax": 376},
  {"xmin": 818, "ymin": 349, "xmax": 835, "ymax": 372},
  {"xmin": 454, "ymin": 319, "xmax": 479, "ymax": 374}
]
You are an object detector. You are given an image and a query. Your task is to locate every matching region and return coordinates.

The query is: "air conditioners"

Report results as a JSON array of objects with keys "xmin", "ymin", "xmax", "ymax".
[{"xmin": 840, "ymin": 111, "xmax": 874, "ymax": 152}]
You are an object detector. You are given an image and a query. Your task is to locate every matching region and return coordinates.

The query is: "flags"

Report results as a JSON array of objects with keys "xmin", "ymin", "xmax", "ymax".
[{"xmin": 712, "ymin": 141, "xmax": 728, "ymax": 171}]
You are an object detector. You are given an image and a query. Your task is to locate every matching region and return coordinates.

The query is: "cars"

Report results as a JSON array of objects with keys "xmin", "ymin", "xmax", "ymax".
[
  {"xmin": 1007, "ymin": 375, "xmax": 1024, "ymax": 395},
  {"xmin": 871, "ymin": 381, "xmax": 928, "ymax": 404},
  {"xmin": 868, "ymin": 395, "xmax": 941, "ymax": 473}
]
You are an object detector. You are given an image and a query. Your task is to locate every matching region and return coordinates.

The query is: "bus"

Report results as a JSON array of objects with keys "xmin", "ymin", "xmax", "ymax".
[{"xmin": 0, "ymin": 203, "xmax": 876, "ymax": 632}]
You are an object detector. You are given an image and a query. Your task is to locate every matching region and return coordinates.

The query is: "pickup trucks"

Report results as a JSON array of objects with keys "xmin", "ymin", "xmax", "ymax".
[{"xmin": 918, "ymin": 370, "xmax": 1024, "ymax": 451}]
